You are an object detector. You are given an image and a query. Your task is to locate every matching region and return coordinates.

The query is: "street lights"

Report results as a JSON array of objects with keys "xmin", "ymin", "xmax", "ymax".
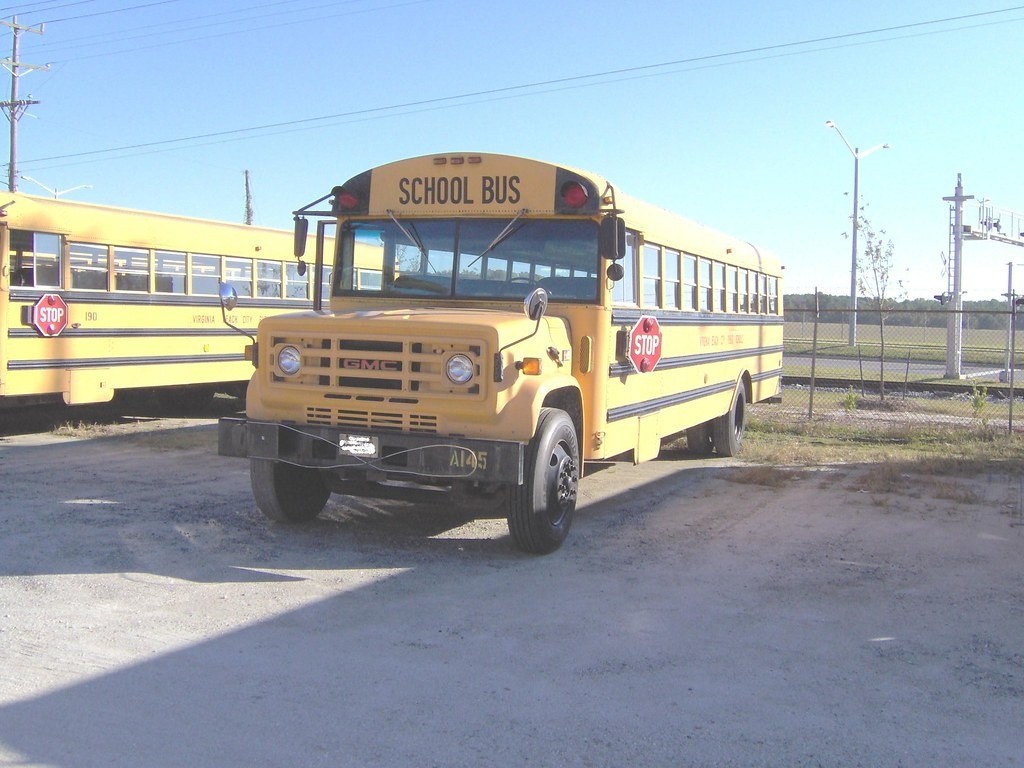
[{"xmin": 826, "ymin": 119, "xmax": 891, "ymax": 347}]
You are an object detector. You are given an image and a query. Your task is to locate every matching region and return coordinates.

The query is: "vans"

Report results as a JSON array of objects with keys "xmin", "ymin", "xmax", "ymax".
[{"xmin": 217, "ymin": 153, "xmax": 785, "ymax": 556}]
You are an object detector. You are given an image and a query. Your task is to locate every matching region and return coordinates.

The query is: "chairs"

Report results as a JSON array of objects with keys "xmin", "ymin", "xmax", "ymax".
[
  {"xmin": 392, "ymin": 275, "xmax": 451, "ymax": 297},
  {"xmin": 535, "ymin": 277, "xmax": 597, "ymax": 301}
]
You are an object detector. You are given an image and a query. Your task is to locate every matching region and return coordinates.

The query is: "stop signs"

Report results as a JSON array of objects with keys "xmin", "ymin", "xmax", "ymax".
[
  {"xmin": 33, "ymin": 294, "xmax": 68, "ymax": 337},
  {"xmin": 626, "ymin": 313, "xmax": 665, "ymax": 374}
]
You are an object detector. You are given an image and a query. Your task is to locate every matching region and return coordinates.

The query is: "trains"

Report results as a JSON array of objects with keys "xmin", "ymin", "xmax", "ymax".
[{"xmin": 0, "ymin": 189, "xmax": 401, "ymax": 405}]
